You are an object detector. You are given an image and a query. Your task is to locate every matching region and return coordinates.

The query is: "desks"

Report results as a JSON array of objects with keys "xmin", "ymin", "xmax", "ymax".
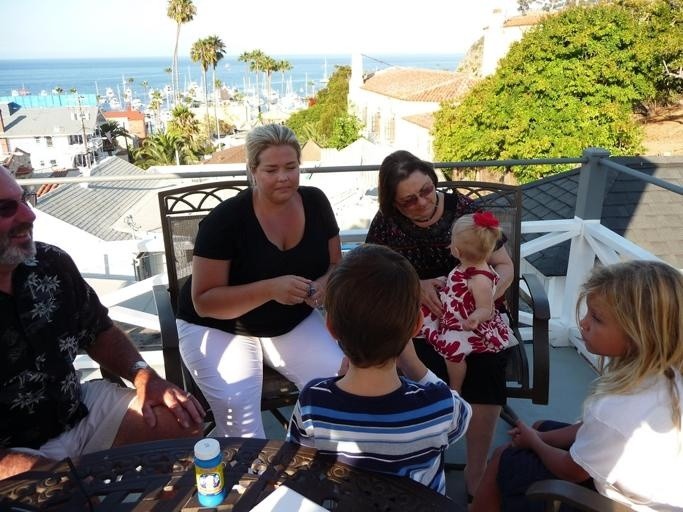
[{"xmin": 0, "ymin": 438, "xmax": 467, "ymax": 512}]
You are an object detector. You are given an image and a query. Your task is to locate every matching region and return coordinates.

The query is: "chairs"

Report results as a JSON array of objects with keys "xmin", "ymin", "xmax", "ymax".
[
  {"xmin": 152, "ymin": 181, "xmax": 300, "ymax": 437},
  {"xmin": 525, "ymin": 479, "xmax": 638, "ymax": 512},
  {"xmin": 434, "ymin": 182, "xmax": 550, "ymax": 470}
]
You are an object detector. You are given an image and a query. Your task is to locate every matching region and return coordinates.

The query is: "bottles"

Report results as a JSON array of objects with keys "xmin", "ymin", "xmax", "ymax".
[
  {"xmin": 307, "ymin": 282, "xmax": 327, "ymax": 321},
  {"xmin": 192, "ymin": 436, "xmax": 227, "ymax": 509}
]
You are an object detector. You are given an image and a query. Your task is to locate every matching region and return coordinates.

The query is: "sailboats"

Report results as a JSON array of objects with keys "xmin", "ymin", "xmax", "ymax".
[{"xmin": 5, "ymin": 59, "xmax": 335, "ymax": 133}]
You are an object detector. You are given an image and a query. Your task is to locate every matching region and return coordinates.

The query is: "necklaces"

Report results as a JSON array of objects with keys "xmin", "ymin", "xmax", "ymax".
[{"xmin": 408, "ymin": 190, "xmax": 440, "ymax": 222}]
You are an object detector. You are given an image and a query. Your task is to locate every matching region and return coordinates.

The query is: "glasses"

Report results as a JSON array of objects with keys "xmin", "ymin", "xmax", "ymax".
[
  {"xmin": 0, "ymin": 192, "xmax": 37, "ymax": 217},
  {"xmin": 393, "ymin": 182, "xmax": 433, "ymax": 207}
]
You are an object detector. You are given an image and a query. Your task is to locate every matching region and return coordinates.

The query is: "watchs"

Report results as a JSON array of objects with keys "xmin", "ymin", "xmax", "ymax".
[{"xmin": 129, "ymin": 360, "xmax": 150, "ymax": 380}]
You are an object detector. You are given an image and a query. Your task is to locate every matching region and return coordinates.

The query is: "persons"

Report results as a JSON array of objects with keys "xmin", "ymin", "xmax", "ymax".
[
  {"xmin": 365, "ymin": 149, "xmax": 507, "ymax": 502},
  {"xmin": 284, "ymin": 242, "xmax": 474, "ymax": 498},
  {"xmin": 0, "ymin": 163, "xmax": 212, "ymax": 483},
  {"xmin": 465, "ymin": 255, "xmax": 683, "ymax": 512},
  {"xmin": 175, "ymin": 122, "xmax": 344, "ymax": 440},
  {"xmin": 411, "ymin": 209, "xmax": 521, "ymax": 395}
]
